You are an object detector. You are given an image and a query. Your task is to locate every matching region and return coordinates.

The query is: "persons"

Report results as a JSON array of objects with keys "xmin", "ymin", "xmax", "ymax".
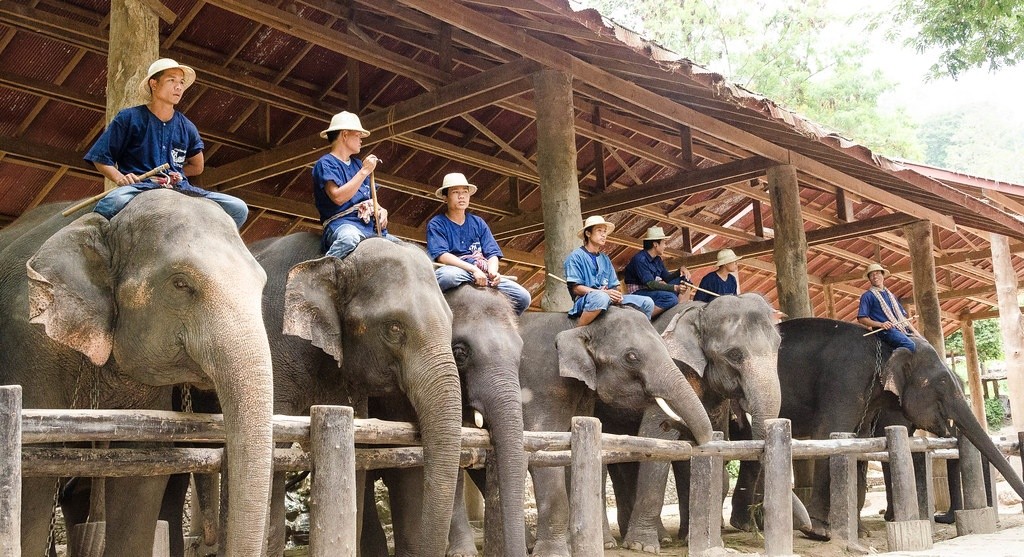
[
  {"xmin": 427, "ymin": 171, "xmax": 532, "ymax": 316},
  {"xmin": 624, "ymin": 226, "xmax": 691, "ymax": 319},
  {"xmin": 564, "ymin": 216, "xmax": 655, "ymax": 326},
  {"xmin": 309, "ymin": 110, "xmax": 404, "ymax": 259},
  {"xmin": 692, "ymin": 250, "xmax": 737, "ymax": 304},
  {"xmin": 83, "ymin": 58, "xmax": 248, "ymax": 234},
  {"xmin": 856, "ymin": 263, "xmax": 916, "ymax": 352}
]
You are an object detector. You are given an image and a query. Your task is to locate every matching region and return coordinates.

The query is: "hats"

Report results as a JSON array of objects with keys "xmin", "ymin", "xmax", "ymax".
[
  {"xmin": 863, "ymin": 264, "xmax": 890, "ymax": 280},
  {"xmin": 640, "ymin": 227, "xmax": 671, "ymax": 240},
  {"xmin": 713, "ymin": 249, "xmax": 743, "ymax": 266},
  {"xmin": 320, "ymin": 111, "xmax": 370, "ymax": 139},
  {"xmin": 577, "ymin": 215, "xmax": 615, "ymax": 240},
  {"xmin": 139, "ymin": 58, "xmax": 196, "ymax": 96},
  {"xmin": 435, "ymin": 173, "xmax": 477, "ymax": 199}
]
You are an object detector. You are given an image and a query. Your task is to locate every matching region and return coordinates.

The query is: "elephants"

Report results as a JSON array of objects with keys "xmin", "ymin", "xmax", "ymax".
[{"xmin": 0, "ymin": 187, "xmax": 1024, "ymax": 557}]
